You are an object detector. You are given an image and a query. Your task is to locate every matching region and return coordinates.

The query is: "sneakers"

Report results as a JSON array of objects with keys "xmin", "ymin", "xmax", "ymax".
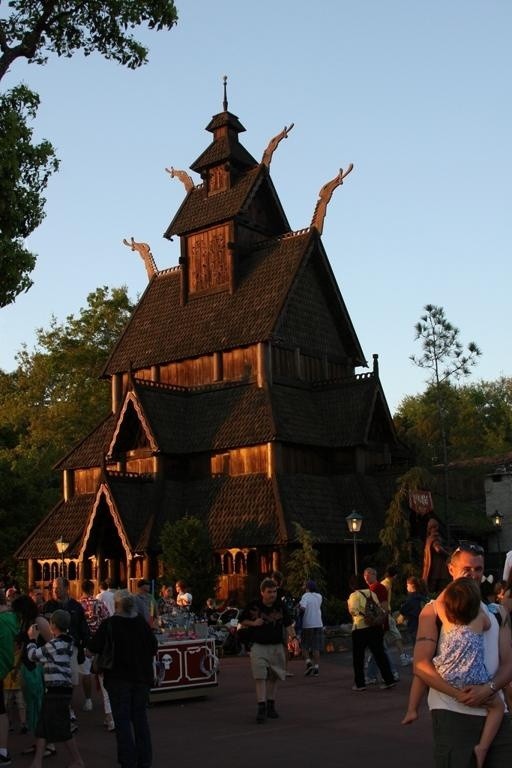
[
  {"xmin": 0, "ymin": 751, "xmax": 13, "ymax": 766},
  {"xmin": 82, "ymin": 701, "xmax": 93, "ymax": 711},
  {"xmin": 400, "ymin": 656, "xmax": 413, "ymax": 668},
  {"xmin": 8, "ymin": 709, "xmax": 80, "ymax": 759},
  {"xmin": 304, "ymin": 662, "xmax": 314, "ymax": 676},
  {"xmin": 286, "ymin": 672, "xmax": 296, "ymax": 677},
  {"xmin": 313, "ymin": 667, "xmax": 320, "ymax": 677},
  {"xmin": 351, "ymin": 663, "xmax": 400, "ymax": 692},
  {"xmin": 103, "ymin": 717, "xmax": 116, "ymax": 731},
  {"xmin": 256, "ymin": 708, "xmax": 265, "ymax": 724},
  {"xmin": 267, "ymin": 706, "xmax": 278, "ymax": 718}
]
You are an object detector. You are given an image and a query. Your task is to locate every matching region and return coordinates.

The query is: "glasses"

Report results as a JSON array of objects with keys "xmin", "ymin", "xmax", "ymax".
[{"xmin": 450, "ymin": 539, "xmax": 485, "ymax": 557}]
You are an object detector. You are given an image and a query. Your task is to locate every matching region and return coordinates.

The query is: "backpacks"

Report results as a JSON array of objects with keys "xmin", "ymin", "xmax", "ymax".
[{"xmin": 358, "ymin": 590, "xmax": 385, "ymax": 627}]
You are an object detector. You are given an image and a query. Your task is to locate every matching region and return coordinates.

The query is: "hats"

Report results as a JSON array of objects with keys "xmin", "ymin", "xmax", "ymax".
[{"xmin": 306, "ymin": 581, "xmax": 317, "ymax": 591}]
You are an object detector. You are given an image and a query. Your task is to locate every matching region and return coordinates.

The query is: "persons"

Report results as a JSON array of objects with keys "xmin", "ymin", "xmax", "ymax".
[
  {"xmin": 1, "ymin": 570, "xmax": 192, "ymax": 768},
  {"xmin": 347, "ymin": 564, "xmax": 426, "ymax": 692},
  {"xmin": 402, "ymin": 542, "xmax": 510, "ymax": 767},
  {"xmin": 207, "ymin": 570, "xmax": 322, "ymax": 723}
]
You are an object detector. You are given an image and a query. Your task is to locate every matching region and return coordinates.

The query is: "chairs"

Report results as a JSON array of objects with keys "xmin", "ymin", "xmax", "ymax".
[{"xmin": 213, "ymin": 607, "xmax": 239, "ymax": 656}]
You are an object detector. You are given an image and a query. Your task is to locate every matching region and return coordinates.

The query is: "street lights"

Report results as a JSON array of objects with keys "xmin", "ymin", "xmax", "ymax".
[
  {"xmin": 345, "ymin": 510, "xmax": 366, "ymax": 576},
  {"xmin": 54, "ymin": 536, "xmax": 71, "ymax": 577},
  {"xmin": 491, "ymin": 510, "xmax": 505, "ymax": 564}
]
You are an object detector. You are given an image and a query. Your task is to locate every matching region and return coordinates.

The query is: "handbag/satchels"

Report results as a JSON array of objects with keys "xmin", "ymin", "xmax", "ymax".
[{"xmin": 92, "ymin": 639, "xmax": 115, "ymax": 675}]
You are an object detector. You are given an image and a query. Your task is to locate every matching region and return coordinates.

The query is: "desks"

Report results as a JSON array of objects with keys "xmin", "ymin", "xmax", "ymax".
[{"xmin": 150, "ymin": 634, "xmax": 218, "ymax": 700}]
